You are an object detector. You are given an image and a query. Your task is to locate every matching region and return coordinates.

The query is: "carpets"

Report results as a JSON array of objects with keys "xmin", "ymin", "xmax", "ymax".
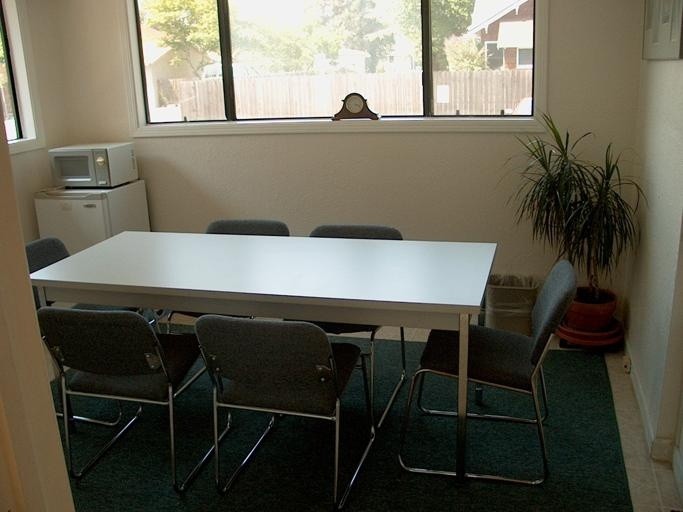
[{"xmin": 50, "ymin": 318, "xmax": 634, "ymax": 512}]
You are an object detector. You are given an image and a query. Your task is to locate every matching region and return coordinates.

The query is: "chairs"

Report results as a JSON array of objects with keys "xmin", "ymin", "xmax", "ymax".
[
  {"xmin": 25, "ymin": 236, "xmax": 164, "ymax": 426},
  {"xmin": 194, "ymin": 312, "xmax": 378, "ymax": 512},
  {"xmin": 159, "ymin": 220, "xmax": 289, "ymax": 402},
  {"xmin": 399, "ymin": 258, "xmax": 579, "ymax": 489},
  {"xmin": 36, "ymin": 306, "xmax": 234, "ymax": 494},
  {"xmin": 277, "ymin": 224, "xmax": 407, "ymax": 429}
]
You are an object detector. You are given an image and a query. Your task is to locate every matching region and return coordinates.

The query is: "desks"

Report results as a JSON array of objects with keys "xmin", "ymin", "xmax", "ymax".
[{"xmin": 28, "ymin": 229, "xmax": 498, "ymax": 488}]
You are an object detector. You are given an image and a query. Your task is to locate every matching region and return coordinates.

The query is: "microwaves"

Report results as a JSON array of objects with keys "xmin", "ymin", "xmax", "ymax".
[{"xmin": 48, "ymin": 143, "xmax": 140, "ymax": 189}]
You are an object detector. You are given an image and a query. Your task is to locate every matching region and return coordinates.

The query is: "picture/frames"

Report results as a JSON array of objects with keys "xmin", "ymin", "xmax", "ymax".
[{"xmin": 640, "ymin": 0, "xmax": 683, "ymax": 62}]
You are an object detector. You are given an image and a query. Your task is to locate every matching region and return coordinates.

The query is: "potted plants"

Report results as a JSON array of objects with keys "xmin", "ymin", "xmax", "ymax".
[{"xmin": 487, "ymin": 103, "xmax": 649, "ymax": 349}]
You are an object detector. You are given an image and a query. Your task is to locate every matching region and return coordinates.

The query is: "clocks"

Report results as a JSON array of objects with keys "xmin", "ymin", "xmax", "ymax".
[{"xmin": 329, "ymin": 92, "xmax": 380, "ymax": 122}]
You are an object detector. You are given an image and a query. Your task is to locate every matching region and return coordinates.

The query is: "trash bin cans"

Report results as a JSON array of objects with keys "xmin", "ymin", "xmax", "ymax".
[{"xmin": 485, "ymin": 274, "xmax": 539, "ymax": 337}]
